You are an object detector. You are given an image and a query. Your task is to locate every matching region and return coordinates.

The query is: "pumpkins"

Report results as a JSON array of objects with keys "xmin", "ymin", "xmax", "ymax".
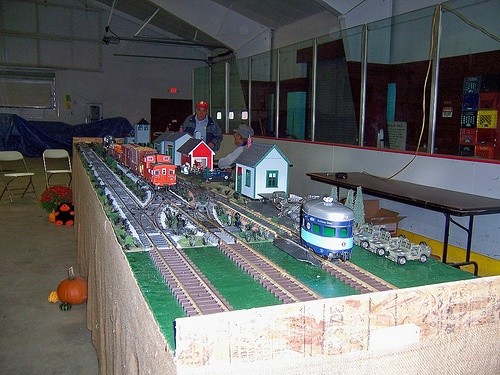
[
  {"xmin": 47, "ymin": 290, "xmax": 58, "ymax": 303},
  {"xmin": 57, "ymin": 266, "xmax": 88, "ymax": 305},
  {"xmin": 49, "ymin": 209, "xmax": 57, "ymax": 222},
  {"xmin": 59, "ymin": 302, "xmax": 72, "ymax": 311}
]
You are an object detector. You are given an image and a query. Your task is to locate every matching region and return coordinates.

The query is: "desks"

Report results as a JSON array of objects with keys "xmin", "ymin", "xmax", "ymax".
[
  {"xmin": 73, "ymin": 137, "xmax": 500, "ymax": 375},
  {"xmin": 306, "ymin": 172, "xmax": 500, "ymax": 277}
]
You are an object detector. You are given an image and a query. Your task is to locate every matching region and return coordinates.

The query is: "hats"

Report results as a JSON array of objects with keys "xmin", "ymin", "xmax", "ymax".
[
  {"xmin": 196, "ymin": 100, "xmax": 210, "ymax": 109},
  {"xmin": 232, "ymin": 123, "xmax": 254, "ymax": 139}
]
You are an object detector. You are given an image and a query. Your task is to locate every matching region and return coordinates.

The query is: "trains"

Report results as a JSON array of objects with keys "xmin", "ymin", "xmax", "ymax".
[{"xmin": 107, "ymin": 144, "xmax": 176, "ymax": 191}]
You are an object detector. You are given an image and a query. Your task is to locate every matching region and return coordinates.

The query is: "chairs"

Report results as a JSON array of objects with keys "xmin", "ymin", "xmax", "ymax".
[
  {"xmin": 0, "ymin": 151, "xmax": 37, "ymax": 204},
  {"xmin": 42, "ymin": 148, "xmax": 73, "ymax": 187}
]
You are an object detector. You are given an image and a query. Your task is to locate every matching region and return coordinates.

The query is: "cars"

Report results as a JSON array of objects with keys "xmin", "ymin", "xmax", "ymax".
[
  {"xmin": 201, "ymin": 169, "xmax": 231, "ymax": 181},
  {"xmin": 353, "ymin": 224, "xmax": 431, "ymax": 265}
]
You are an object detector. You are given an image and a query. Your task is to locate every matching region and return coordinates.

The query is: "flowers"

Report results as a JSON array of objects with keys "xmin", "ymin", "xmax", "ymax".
[{"xmin": 38, "ymin": 185, "xmax": 75, "ymax": 227}]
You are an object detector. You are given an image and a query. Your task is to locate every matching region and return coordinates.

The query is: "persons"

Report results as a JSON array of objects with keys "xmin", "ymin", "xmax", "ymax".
[
  {"xmin": 181, "ymin": 100, "xmax": 223, "ymax": 153},
  {"xmin": 218, "ymin": 124, "xmax": 254, "ymax": 169}
]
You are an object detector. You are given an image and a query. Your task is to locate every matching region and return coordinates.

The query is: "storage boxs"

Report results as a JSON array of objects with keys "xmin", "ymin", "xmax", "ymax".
[
  {"xmin": 362, "ymin": 199, "xmax": 407, "ymax": 236},
  {"xmin": 458, "ymin": 73, "xmax": 500, "ymax": 160}
]
